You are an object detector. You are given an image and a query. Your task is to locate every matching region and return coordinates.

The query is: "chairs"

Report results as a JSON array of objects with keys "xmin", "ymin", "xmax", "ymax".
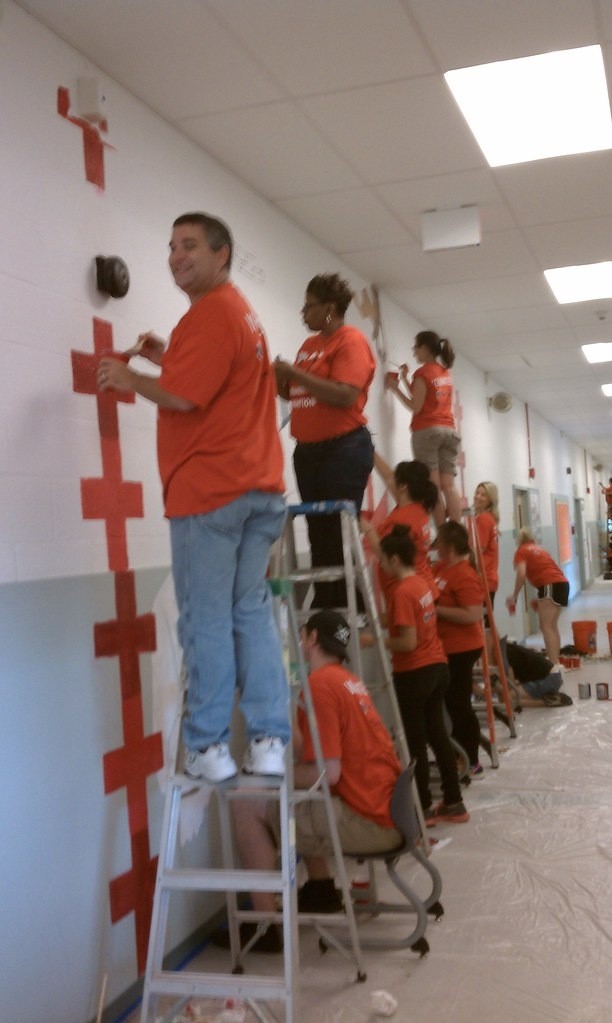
[{"xmin": 318, "ymin": 760, "xmax": 445, "ymax": 959}]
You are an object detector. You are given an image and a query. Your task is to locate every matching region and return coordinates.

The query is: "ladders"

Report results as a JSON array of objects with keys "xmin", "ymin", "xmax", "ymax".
[
  {"xmin": 281, "ymin": 502, "xmax": 430, "ymax": 860},
  {"xmin": 142, "ymin": 580, "xmax": 368, "ymax": 1023},
  {"xmin": 448, "ymin": 509, "xmax": 517, "ymax": 769}
]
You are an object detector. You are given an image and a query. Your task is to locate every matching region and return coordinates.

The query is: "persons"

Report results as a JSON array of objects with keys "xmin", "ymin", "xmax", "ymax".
[
  {"xmin": 272, "ymin": 271, "xmax": 377, "ymax": 631},
  {"xmin": 466, "ymin": 481, "xmax": 502, "ymax": 633},
  {"xmin": 217, "ymin": 610, "xmax": 405, "ymax": 950},
  {"xmin": 506, "ymin": 526, "xmax": 569, "ymax": 675},
  {"xmin": 95, "ymin": 211, "xmax": 295, "ymax": 783},
  {"xmin": 429, "ymin": 519, "xmax": 486, "ymax": 781},
  {"xmin": 469, "ymin": 630, "xmax": 574, "ymax": 706},
  {"xmin": 384, "ymin": 331, "xmax": 466, "ymax": 547},
  {"xmin": 359, "ymin": 525, "xmax": 470, "ymax": 828},
  {"xmin": 369, "ymin": 445, "xmax": 441, "ymax": 628},
  {"xmin": 598, "ymin": 477, "xmax": 612, "ymax": 580}
]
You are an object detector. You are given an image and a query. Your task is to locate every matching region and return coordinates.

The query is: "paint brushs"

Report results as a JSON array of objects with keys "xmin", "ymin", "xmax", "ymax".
[{"xmin": 388, "ymin": 360, "xmax": 411, "ymax": 374}]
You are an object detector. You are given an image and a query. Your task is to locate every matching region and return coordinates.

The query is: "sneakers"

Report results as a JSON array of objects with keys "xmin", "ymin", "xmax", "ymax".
[
  {"xmin": 242, "ymin": 737, "xmax": 285, "ymax": 776},
  {"xmin": 423, "ymin": 810, "xmax": 435, "ymax": 826},
  {"xmin": 440, "ymin": 775, "xmax": 471, "ymax": 791},
  {"xmin": 182, "ymin": 743, "xmax": 237, "ymax": 782},
  {"xmin": 432, "ymin": 801, "xmax": 468, "ymax": 822},
  {"xmin": 214, "ymin": 922, "xmax": 284, "ymax": 955},
  {"xmin": 468, "ymin": 762, "xmax": 483, "ymax": 780},
  {"xmin": 298, "ymin": 877, "xmax": 342, "ymax": 915}
]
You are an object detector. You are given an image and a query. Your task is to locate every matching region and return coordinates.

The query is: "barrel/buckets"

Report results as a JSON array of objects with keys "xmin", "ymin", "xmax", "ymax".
[
  {"xmin": 607, "ymin": 622, "xmax": 612, "ymax": 657},
  {"xmin": 572, "ymin": 621, "xmax": 597, "ymax": 654}
]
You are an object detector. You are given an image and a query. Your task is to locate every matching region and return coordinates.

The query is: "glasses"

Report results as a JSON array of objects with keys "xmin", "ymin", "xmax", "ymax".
[
  {"xmin": 412, "ymin": 344, "xmax": 424, "ymax": 352},
  {"xmin": 304, "ymin": 302, "xmax": 324, "ymax": 311}
]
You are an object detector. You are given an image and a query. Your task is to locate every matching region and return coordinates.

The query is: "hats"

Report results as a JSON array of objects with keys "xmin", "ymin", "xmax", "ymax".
[{"xmin": 307, "ymin": 611, "xmax": 352, "ymax": 646}]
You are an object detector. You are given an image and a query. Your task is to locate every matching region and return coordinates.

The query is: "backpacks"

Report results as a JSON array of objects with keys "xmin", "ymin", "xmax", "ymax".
[{"xmin": 506, "ymin": 643, "xmax": 554, "ymax": 682}]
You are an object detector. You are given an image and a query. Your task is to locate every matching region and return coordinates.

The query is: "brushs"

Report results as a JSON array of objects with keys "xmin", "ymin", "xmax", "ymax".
[{"xmin": 125, "ymin": 329, "xmax": 155, "ymax": 356}]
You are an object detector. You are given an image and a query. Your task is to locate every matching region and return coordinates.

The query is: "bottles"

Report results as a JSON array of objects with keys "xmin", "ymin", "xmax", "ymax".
[
  {"xmin": 578, "ymin": 682, "xmax": 591, "ymax": 699},
  {"xmin": 596, "ymin": 683, "xmax": 608, "ymax": 700}
]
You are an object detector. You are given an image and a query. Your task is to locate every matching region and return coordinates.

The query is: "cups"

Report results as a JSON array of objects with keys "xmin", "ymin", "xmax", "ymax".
[
  {"xmin": 387, "ymin": 373, "xmax": 398, "ymax": 379},
  {"xmin": 352, "ymin": 876, "xmax": 373, "ymax": 904}
]
